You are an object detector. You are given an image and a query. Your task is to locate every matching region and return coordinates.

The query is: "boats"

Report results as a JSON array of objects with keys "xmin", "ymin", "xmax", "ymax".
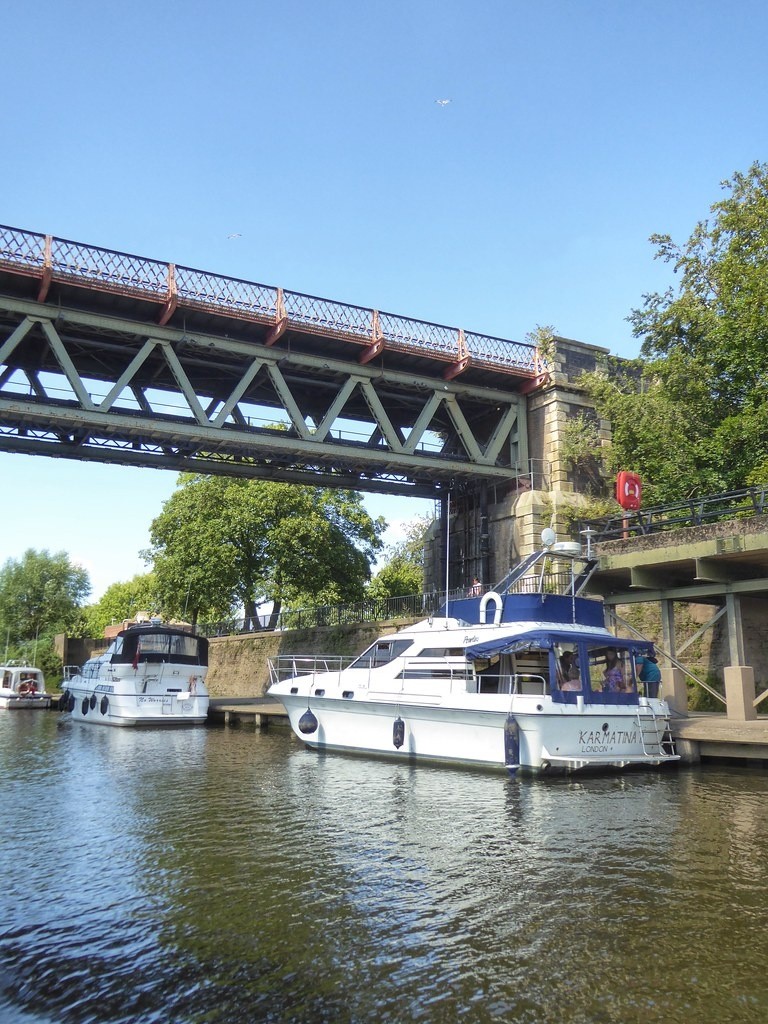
[
  {"xmin": 0, "ymin": 665, "xmax": 51, "ymax": 710},
  {"xmin": 62, "ymin": 619, "xmax": 211, "ymax": 729},
  {"xmin": 268, "ymin": 528, "xmax": 687, "ymax": 777}
]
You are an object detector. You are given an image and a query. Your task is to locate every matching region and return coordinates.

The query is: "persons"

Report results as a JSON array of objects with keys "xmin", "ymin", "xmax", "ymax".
[
  {"xmin": 560, "ymin": 651, "xmax": 575, "ymax": 681},
  {"xmin": 601, "ymin": 647, "xmax": 626, "ymax": 692},
  {"xmin": 466, "ymin": 578, "xmax": 483, "ymax": 596},
  {"xmin": 562, "ymin": 666, "xmax": 581, "ymax": 691},
  {"xmin": 629, "ymin": 656, "xmax": 661, "ymax": 697}
]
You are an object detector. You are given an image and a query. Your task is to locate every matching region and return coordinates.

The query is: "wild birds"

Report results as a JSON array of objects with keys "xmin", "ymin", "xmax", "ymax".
[
  {"xmin": 226, "ymin": 232, "xmax": 242, "ymax": 239},
  {"xmin": 435, "ymin": 99, "xmax": 452, "ymax": 107}
]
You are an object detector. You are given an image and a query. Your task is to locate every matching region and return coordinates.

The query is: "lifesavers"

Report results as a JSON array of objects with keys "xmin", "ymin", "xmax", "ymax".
[
  {"xmin": 480, "ymin": 591, "xmax": 503, "ymax": 624},
  {"xmin": 624, "ymin": 479, "xmax": 638, "ymax": 498},
  {"xmin": 17, "ymin": 683, "xmax": 30, "ymax": 695}
]
